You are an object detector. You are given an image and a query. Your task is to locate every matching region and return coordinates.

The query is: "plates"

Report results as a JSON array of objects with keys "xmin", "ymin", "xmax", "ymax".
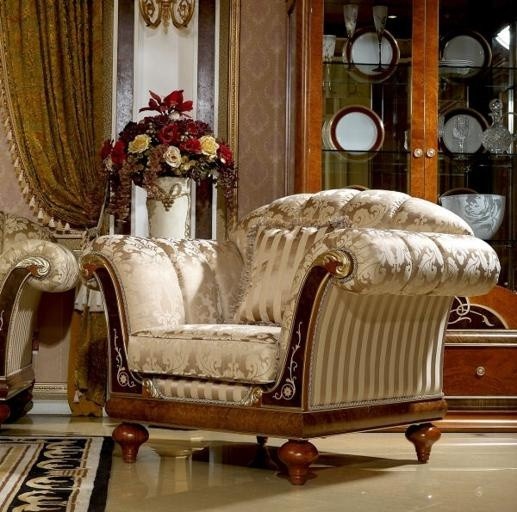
[
  {"xmin": 440, "ymin": 187, "xmax": 477, "ymax": 196},
  {"xmin": 338, "ymin": 26, "xmax": 400, "ymax": 84},
  {"xmin": 440, "ymin": 106, "xmax": 491, "ymax": 159},
  {"xmin": 439, "ymin": 29, "xmax": 492, "ymax": 86},
  {"xmin": 321, "ymin": 103, "xmax": 386, "ymax": 161}
]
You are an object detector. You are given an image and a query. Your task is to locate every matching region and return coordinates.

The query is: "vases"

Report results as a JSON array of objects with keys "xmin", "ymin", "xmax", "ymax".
[{"xmin": 140, "ymin": 174, "xmax": 195, "ymax": 242}]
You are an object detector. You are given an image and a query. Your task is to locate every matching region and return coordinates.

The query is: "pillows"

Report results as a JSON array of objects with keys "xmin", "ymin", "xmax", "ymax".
[{"xmin": 228, "ymin": 214, "xmax": 353, "ymax": 331}]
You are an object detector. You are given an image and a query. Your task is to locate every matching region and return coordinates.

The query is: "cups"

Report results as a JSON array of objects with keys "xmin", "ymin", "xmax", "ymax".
[{"xmin": 323, "ymin": 34, "xmax": 336, "ymax": 62}]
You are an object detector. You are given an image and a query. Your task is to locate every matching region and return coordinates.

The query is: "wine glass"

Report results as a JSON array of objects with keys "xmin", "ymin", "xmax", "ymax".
[
  {"xmin": 453, "ymin": 116, "xmax": 469, "ymax": 161},
  {"xmin": 371, "ymin": 5, "xmax": 388, "ymax": 72},
  {"xmin": 342, "ymin": 3, "xmax": 359, "ymax": 64}
]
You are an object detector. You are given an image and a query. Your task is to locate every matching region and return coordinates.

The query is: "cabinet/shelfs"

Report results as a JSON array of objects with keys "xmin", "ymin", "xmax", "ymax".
[{"xmin": 284, "ymin": 0, "xmax": 516, "ymax": 439}]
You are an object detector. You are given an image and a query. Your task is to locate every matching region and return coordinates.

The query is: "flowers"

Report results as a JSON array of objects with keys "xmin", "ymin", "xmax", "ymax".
[{"xmin": 94, "ymin": 89, "xmax": 237, "ymax": 227}]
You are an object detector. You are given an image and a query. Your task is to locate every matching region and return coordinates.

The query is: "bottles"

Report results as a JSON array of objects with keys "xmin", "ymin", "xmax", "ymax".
[{"xmin": 481, "ymin": 99, "xmax": 517, "ymax": 155}]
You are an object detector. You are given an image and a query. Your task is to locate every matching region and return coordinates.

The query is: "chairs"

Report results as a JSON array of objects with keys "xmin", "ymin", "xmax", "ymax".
[
  {"xmin": 0, "ymin": 211, "xmax": 82, "ymax": 426},
  {"xmin": 74, "ymin": 186, "xmax": 504, "ymax": 489}
]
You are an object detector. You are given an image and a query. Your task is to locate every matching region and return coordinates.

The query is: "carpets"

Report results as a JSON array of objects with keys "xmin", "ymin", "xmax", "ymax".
[{"xmin": 0, "ymin": 432, "xmax": 113, "ymax": 510}]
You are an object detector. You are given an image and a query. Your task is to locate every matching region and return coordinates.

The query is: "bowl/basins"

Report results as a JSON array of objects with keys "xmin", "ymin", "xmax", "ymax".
[{"xmin": 441, "ymin": 192, "xmax": 507, "ymax": 240}]
